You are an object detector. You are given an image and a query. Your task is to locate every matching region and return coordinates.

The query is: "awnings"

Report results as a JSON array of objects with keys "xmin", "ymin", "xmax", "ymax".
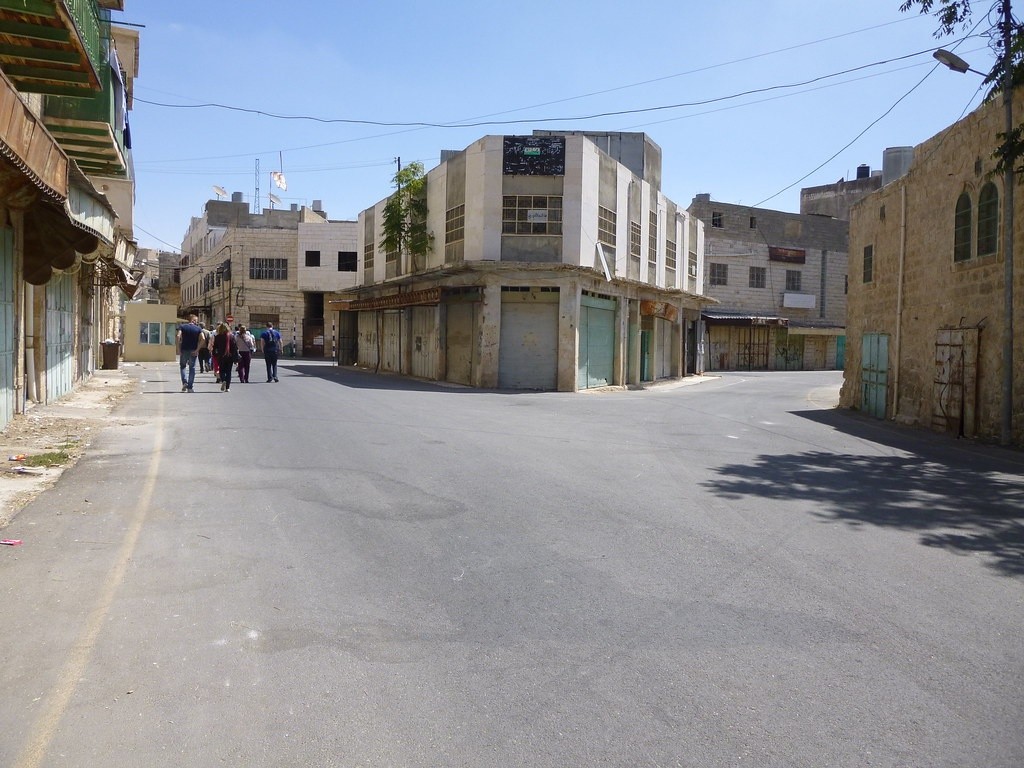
[
  {"xmin": 704, "ymin": 313, "xmax": 789, "ymax": 321},
  {"xmin": 0, "ymin": 72, "xmax": 116, "ymax": 285}
]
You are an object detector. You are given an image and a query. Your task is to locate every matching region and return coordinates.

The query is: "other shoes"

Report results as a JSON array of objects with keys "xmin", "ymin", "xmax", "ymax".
[
  {"xmin": 205, "ymin": 365, "xmax": 209, "ymax": 372},
  {"xmin": 241, "ymin": 380, "xmax": 245, "ymax": 383},
  {"xmin": 220, "ymin": 381, "xmax": 226, "ymax": 391},
  {"xmin": 246, "ymin": 380, "xmax": 250, "ymax": 383},
  {"xmin": 182, "ymin": 383, "xmax": 188, "ymax": 391},
  {"xmin": 225, "ymin": 389, "xmax": 230, "ymax": 392},
  {"xmin": 200, "ymin": 369, "xmax": 203, "ymax": 373},
  {"xmin": 274, "ymin": 377, "xmax": 279, "ymax": 382},
  {"xmin": 216, "ymin": 378, "xmax": 220, "ymax": 383},
  {"xmin": 187, "ymin": 389, "xmax": 194, "ymax": 392},
  {"xmin": 266, "ymin": 378, "xmax": 272, "ymax": 382}
]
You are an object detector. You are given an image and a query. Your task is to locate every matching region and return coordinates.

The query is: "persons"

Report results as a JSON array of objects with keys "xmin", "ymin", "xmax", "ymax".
[
  {"xmin": 175, "ymin": 314, "xmax": 257, "ymax": 392},
  {"xmin": 118, "ymin": 329, "xmax": 123, "ymax": 356},
  {"xmin": 143, "ymin": 326, "xmax": 148, "ymax": 341},
  {"xmin": 260, "ymin": 322, "xmax": 284, "ymax": 383}
]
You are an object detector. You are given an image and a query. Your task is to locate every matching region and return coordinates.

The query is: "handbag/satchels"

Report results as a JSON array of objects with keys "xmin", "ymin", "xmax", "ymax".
[{"xmin": 229, "ymin": 333, "xmax": 240, "ymax": 361}]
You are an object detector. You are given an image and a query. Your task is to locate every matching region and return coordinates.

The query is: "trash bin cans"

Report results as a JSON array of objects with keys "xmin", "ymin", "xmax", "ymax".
[{"xmin": 99, "ymin": 340, "xmax": 122, "ymax": 370}]
